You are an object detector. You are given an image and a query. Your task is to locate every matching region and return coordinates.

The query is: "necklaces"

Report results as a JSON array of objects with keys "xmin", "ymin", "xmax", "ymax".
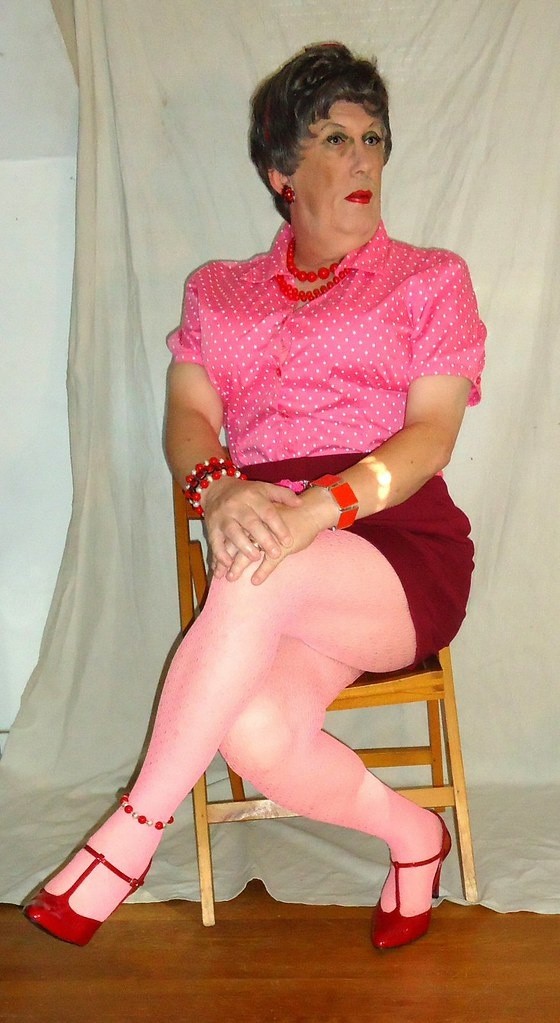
[{"xmin": 272, "ymin": 240, "xmax": 354, "ymax": 301}]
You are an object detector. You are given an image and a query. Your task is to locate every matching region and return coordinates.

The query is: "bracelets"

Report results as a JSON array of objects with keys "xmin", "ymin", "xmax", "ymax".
[
  {"xmin": 182, "ymin": 457, "xmax": 247, "ymax": 518},
  {"xmin": 359, "ymin": 456, "xmax": 392, "ymax": 513},
  {"xmin": 308, "ymin": 474, "xmax": 359, "ymax": 531}
]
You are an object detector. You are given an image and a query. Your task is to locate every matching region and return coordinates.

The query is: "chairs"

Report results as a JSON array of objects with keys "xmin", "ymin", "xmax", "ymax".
[{"xmin": 173, "ymin": 448, "xmax": 480, "ymax": 928}]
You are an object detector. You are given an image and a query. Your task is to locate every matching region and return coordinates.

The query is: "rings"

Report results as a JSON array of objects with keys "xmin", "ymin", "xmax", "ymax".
[{"xmin": 250, "ymin": 539, "xmax": 261, "ymax": 551}]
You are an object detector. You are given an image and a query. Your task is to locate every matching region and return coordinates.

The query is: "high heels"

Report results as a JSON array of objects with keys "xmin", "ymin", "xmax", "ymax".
[
  {"xmin": 23, "ymin": 843, "xmax": 153, "ymax": 947},
  {"xmin": 370, "ymin": 808, "xmax": 451, "ymax": 949}
]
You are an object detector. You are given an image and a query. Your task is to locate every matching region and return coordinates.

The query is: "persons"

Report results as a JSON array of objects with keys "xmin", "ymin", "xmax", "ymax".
[{"xmin": 23, "ymin": 42, "xmax": 488, "ymax": 950}]
